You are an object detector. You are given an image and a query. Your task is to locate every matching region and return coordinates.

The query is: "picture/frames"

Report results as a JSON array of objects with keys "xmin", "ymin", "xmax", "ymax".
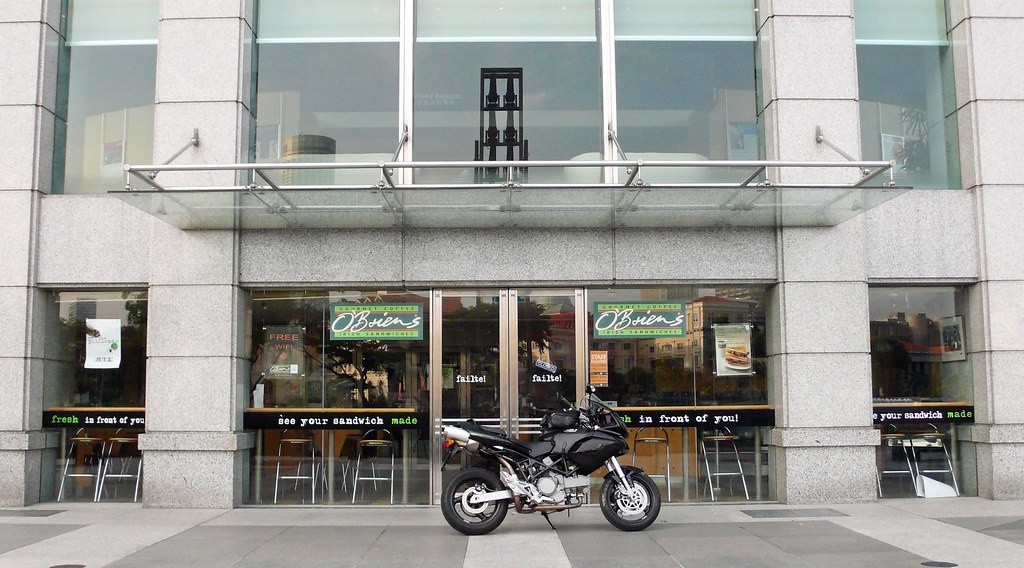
[{"xmin": 938, "ymin": 315, "xmax": 968, "ymax": 363}]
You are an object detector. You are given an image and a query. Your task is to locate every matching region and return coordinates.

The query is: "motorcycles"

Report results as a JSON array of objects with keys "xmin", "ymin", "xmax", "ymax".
[{"xmin": 441, "ymin": 383, "xmax": 661, "ymax": 535}]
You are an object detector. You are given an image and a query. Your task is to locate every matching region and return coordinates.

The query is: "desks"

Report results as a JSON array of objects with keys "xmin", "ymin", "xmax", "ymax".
[
  {"xmin": 873, "ymin": 402, "xmax": 971, "ymax": 494},
  {"xmin": 246, "ymin": 408, "xmax": 416, "ymax": 504},
  {"xmin": 610, "ymin": 405, "xmax": 776, "ymax": 501},
  {"xmin": 49, "ymin": 406, "xmax": 145, "ymax": 499}
]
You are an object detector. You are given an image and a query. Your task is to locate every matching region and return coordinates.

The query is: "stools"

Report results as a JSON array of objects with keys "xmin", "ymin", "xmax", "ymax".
[
  {"xmin": 875, "ymin": 422, "xmax": 917, "ymax": 498},
  {"xmin": 910, "ymin": 423, "xmax": 960, "ymax": 498},
  {"xmin": 273, "ymin": 429, "xmax": 328, "ymax": 505},
  {"xmin": 352, "ymin": 428, "xmax": 395, "ymax": 505},
  {"xmin": 702, "ymin": 426, "xmax": 751, "ymax": 502},
  {"xmin": 632, "ymin": 428, "xmax": 672, "ymax": 503},
  {"xmin": 98, "ymin": 428, "xmax": 143, "ymax": 503}
]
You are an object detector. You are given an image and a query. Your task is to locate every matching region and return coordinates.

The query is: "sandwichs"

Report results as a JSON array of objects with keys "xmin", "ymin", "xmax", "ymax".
[{"xmin": 725, "ymin": 345, "xmax": 749, "ymax": 367}]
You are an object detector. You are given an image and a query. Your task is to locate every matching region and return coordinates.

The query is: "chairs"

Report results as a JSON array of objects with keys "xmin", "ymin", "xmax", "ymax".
[{"xmin": 57, "ymin": 427, "xmax": 105, "ymax": 502}]
